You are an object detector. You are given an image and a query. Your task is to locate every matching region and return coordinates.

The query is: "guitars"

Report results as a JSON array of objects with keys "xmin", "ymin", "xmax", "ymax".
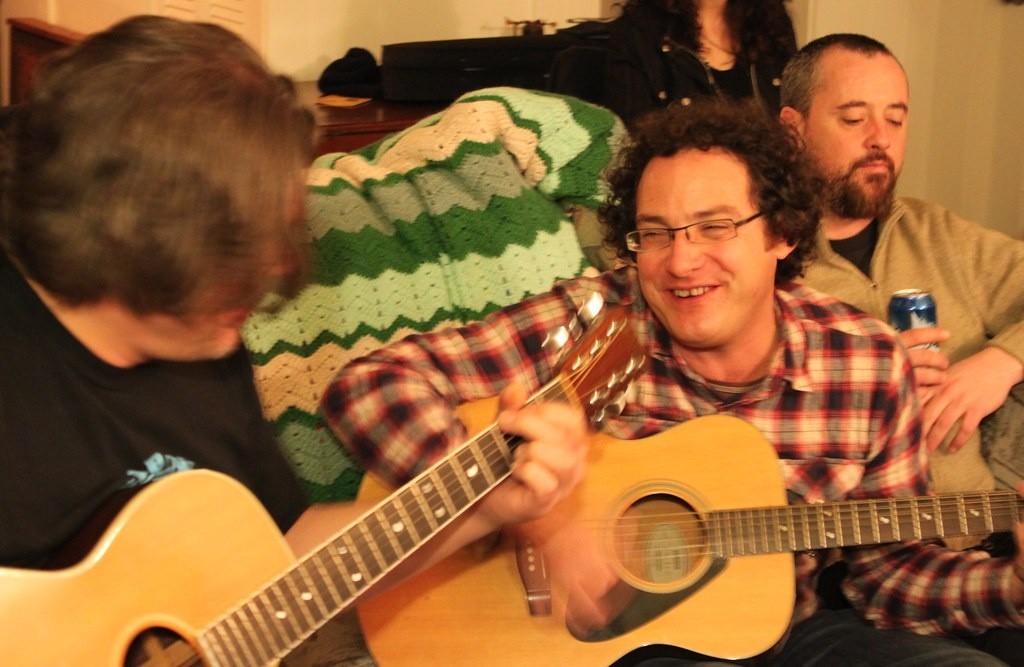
[
  {"xmin": 351, "ymin": 411, "xmax": 1023, "ymax": 667},
  {"xmin": 0, "ymin": 291, "xmax": 647, "ymax": 666}
]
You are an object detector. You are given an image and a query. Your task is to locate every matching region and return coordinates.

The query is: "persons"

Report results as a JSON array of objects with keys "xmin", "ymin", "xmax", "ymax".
[
  {"xmin": 318, "ymin": 99, "xmax": 1024, "ymax": 667},
  {"xmin": 0, "ymin": 16, "xmax": 589, "ymax": 667},
  {"xmin": 777, "ymin": 34, "xmax": 1024, "ymax": 559},
  {"xmin": 602, "ymin": 0, "xmax": 797, "ymax": 139}
]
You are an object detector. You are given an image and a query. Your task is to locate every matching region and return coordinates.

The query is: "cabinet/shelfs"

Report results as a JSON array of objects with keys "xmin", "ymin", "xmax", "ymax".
[{"xmin": 295, "ymin": 80, "xmax": 446, "ymax": 166}]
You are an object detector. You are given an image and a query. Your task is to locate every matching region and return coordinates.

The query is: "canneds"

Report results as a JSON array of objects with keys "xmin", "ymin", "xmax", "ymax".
[{"xmin": 887, "ymin": 288, "xmax": 939, "ymax": 352}]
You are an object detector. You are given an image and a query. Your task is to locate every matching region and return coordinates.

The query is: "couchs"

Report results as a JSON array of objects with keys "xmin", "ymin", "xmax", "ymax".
[{"xmin": 240, "ymin": 86, "xmax": 633, "ymax": 503}]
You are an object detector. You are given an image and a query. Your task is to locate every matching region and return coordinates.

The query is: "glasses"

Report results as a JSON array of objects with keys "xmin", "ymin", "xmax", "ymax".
[{"xmin": 626, "ymin": 211, "xmax": 765, "ymax": 253}]
